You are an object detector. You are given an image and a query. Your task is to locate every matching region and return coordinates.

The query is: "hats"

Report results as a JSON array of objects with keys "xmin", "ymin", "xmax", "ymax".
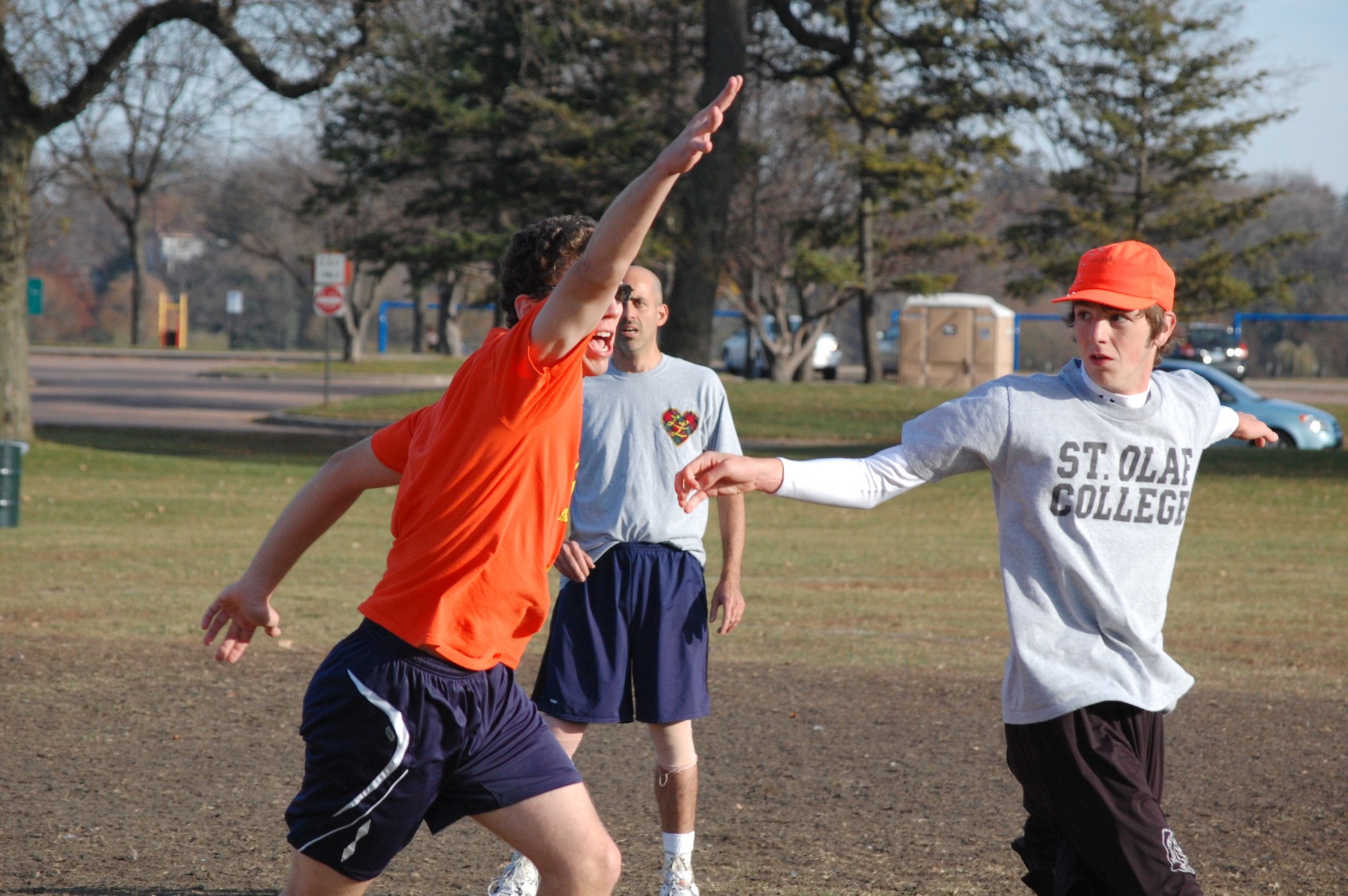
[{"xmin": 1051, "ymin": 238, "xmax": 1175, "ymax": 312}]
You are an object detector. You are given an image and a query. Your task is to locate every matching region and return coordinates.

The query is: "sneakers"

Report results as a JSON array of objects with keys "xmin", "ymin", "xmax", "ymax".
[
  {"xmin": 660, "ymin": 858, "xmax": 699, "ymax": 896},
  {"xmin": 487, "ymin": 854, "xmax": 537, "ymax": 896}
]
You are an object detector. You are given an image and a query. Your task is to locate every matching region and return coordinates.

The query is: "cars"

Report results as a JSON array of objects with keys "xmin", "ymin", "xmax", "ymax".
[
  {"xmin": 717, "ymin": 315, "xmax": 841, "ymax": 380},
  {"xmin": 1152, "ymin": 357, "xmax": 1344, "ymax": 450}
]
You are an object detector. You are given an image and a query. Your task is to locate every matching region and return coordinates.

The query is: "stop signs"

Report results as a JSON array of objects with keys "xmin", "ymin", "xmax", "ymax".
[{"xmin": 312, "ymin": 284, "xmax": 346, "ymax": 318}]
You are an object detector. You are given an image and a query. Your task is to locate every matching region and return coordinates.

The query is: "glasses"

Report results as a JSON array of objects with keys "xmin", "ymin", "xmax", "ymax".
[{"xmin": 615, "ymin": 283, "xmax": 632, "ymax": 303}]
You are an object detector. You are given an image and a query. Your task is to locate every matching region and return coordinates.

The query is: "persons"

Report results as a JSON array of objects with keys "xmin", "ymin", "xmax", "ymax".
[
  {"xmin": 670, "ymin": 240, "xmax": 1280, "ymax": 896},
  {"xmin": 199, "ymin": 68, "xmax": 748, "ymax": 896},
  {"xmin": 480, "ymin": 265, "xmax": 751, "ymax": 896}
]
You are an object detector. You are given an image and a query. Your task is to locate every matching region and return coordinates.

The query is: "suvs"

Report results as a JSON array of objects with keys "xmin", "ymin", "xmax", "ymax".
[{"xmin": 1162, "ymin": 322, "xmax": 1249, "ymax": 382}]
